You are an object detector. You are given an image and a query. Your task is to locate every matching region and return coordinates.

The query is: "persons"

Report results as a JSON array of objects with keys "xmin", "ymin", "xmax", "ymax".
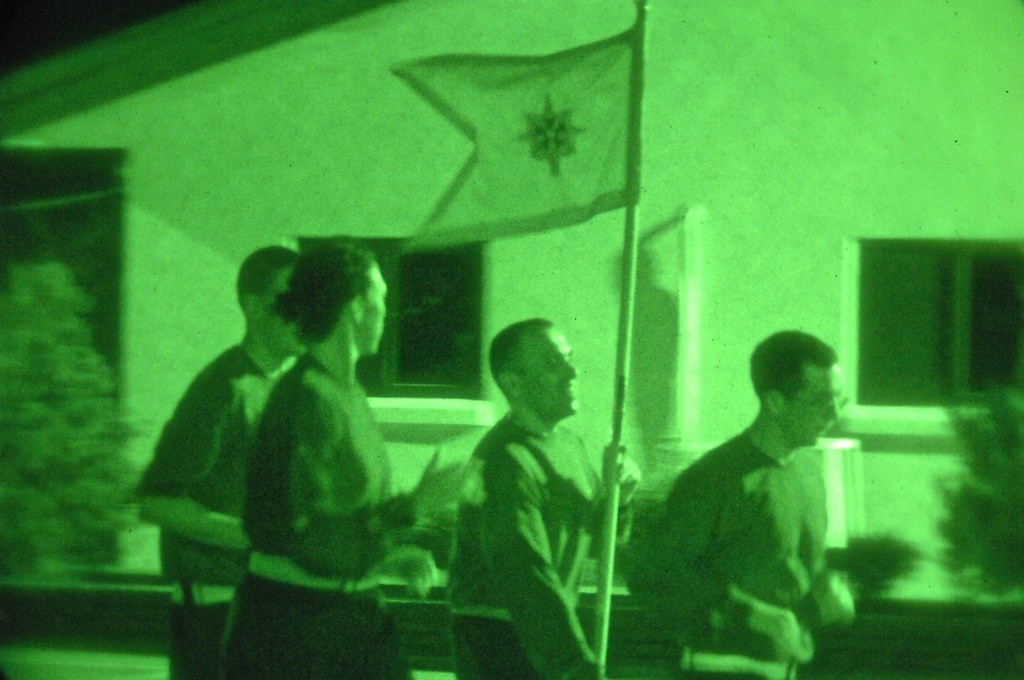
[
  {"xmin": 441, "ymin": 303, "xmax": 641, "ymax": 680},
  {"xmin": 618, "ymin": 328, "xmax": 863, "ymax": 678},
  {"xmin": 138, "ymin": 245, "xmax": 309, "ymax": 678},
  {"xmin": 220, "ymin": 237, "xmax": 482, "ymax": 680}
]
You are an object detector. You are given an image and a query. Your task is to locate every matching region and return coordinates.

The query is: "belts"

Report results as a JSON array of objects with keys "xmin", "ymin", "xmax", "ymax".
[
  {"xmin": 171, "ymin": 582, "xmax": 238, "ymax": 606},
  {"xmin": 249, "ymin": 550, "xmax": 379, "ymax": 592},
  {"xmin": 679, "ymin": 646, "xmax": 797, "ymax": 680}
]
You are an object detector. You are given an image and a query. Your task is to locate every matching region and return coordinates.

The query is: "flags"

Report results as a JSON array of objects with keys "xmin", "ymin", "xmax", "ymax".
[{"xmin": 387, "ymin": 23, "xmax": 645, "ymax": 253}]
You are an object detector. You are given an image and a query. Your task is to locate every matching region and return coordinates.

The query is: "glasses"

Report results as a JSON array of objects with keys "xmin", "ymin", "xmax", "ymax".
[{"xmin": 791, "ymin": 388, "xmax": 849, "ymax": 411}]
